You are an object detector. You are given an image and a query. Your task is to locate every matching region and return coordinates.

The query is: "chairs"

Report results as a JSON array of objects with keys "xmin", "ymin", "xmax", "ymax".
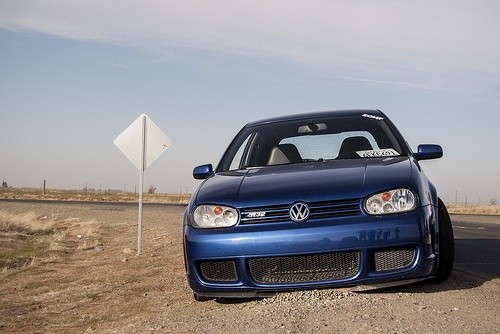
[
  {"xmin": 266, "ymin": 144, "xmax": 303, "ymax": 166},
  {"xmin": 337, "ymin": 136, "xmax": 374, "ymax": 159}
]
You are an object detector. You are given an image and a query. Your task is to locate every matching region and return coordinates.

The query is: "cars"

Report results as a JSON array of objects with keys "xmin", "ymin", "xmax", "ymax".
[{"xmin": 182, "ymin": 110, "xmax": 454, "ymax": 302}]
